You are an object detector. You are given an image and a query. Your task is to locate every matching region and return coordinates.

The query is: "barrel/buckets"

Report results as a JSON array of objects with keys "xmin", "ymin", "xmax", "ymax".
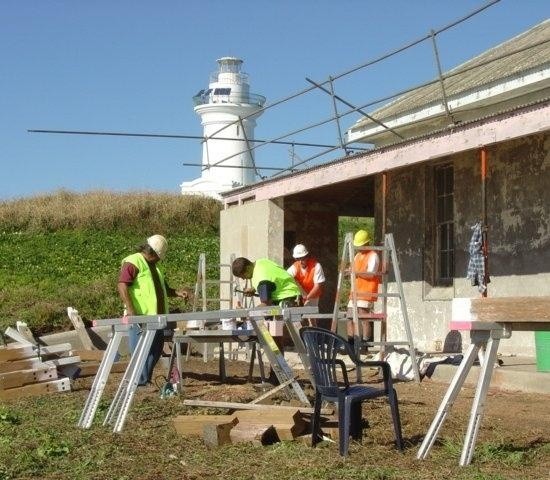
[
  {"xmin": 535, "ymin": 331, "xmax": 550, "ymax": 371},
  {"xmin": 221, "ymin": 318, "xmax": 236, "ymax": 331}
]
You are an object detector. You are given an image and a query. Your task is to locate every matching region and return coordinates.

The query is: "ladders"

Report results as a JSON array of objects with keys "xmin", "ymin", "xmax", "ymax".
[
  {"xmin": 330, "ymin": 232, "xmax": 421, "ymax": 384},
  {"xmin": 186, "ymin": 253, "xmax": 251, "ymax": 363}
]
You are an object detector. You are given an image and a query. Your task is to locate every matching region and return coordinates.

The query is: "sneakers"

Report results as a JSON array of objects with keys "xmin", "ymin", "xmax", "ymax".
[{"xmin": 137, "ymin": 382, "xmax": 159, "ymax": 394}]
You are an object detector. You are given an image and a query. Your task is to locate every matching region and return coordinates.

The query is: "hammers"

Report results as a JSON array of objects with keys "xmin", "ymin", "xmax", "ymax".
[{"xmin": 233, "ymin": 284, "xmax": 260, "ymax": 297}]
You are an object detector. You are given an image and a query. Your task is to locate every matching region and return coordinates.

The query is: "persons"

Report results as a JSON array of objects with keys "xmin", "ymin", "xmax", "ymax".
[
  {"xmin": 286, "ymin": 243, "xmax": 325, "ymax": 364},
  {"xmin": 338, "ymin": 229, "xmax": 380, "ymax": 355},
  {"xmin": 117, "ymin": 235, "xmax": 189, "ymax": 394},
  {"xmin": 231, "ymin": 256, "xmax": 305, "ymax": 387}
]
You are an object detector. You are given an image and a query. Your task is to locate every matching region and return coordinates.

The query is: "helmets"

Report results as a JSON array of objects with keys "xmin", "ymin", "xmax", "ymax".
[
  {"xmin": 354, "ymin": 229, "xmax": 372, "ymax": 246},
  {"xmin": 147, "ymin": 234, "xmax": 168, "ymax": 260},
  {"xmin": 292, "ymin": 244, "xmax": 309, "ymax": 258}
]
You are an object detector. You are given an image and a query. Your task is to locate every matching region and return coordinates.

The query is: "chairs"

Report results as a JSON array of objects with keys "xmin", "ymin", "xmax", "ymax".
[{"xmin": 298, "ymin": 325, "xmax": 405, "ymax": 457}]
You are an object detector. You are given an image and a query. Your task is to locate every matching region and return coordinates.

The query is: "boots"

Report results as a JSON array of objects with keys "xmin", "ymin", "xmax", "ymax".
[{"xmin": 341, "ymin": 335, "xmax": 372, "ymax": 355}]
